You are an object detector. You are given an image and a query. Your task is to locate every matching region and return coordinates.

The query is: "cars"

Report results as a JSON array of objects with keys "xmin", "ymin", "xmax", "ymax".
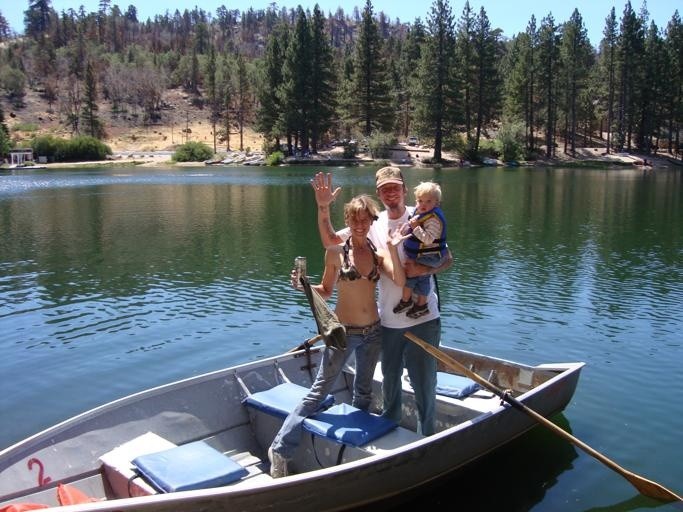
[
  {"xmin": 409, "ymin": 137, "xmax": 419, "ymax": 146},
  {"xmin": 331, "ymin": 139, "xmax": 358, "ymax": 146}
]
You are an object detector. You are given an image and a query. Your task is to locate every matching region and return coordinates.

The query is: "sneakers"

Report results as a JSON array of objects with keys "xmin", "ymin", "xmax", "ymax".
[
  {"xmin": 268, "ymin": 446, "xmax": 293, "ymax": 478},
  {"xmin": 405, "ymin": 302, "xmax": 430, "ymax": 319},
  {"xmin": 393, "ymin": 296, "xmax": 414, "ymax": 315}
]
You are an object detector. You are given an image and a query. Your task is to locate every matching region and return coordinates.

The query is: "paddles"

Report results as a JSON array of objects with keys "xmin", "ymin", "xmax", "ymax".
[{"xmin": 404, "ymin": 331, "xmax": 683, "ymax": 501}]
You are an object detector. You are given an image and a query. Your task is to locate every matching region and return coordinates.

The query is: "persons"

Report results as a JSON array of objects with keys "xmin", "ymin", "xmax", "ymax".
[
  {"xmin": 391, "ymin": 179, "xmax": 448, "ymax": 320},
  {"xmin": 642, "ymin": 159, "xmax": 648, "ymax": 165},
  {"xmin": 414, "ymin": 153, "xmax": 421, "ymax": 168},
  {"xmin": 637, "ymin": 159, "xmax": 642, "ymax": 165},
  {"xmin": 648, "ymin": 161, "xmax": 653, "ymax": 167},
  {"xmin": 457, "ymin": 159, "xmax": 463, "ymax": 169},
  {"xmin": 266, "ymin": 191, "xmax": 414, "ymax": 478},
  {"xmin": 308, "ymin": 165, "xmax": 454, "ymax": 439}
]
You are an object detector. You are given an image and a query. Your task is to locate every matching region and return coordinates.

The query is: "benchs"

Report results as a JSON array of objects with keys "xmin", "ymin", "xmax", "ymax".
[
  {"xmin": 101, "ymin": 432, "xmax": 221, "ymax": 496},
  {"xmin": 344, "ymin": 360, "xmax": 506, "ymax": 436},
  {"xmin": 245, "ymin": 383, "xmax": 425, "ymax": 472}
]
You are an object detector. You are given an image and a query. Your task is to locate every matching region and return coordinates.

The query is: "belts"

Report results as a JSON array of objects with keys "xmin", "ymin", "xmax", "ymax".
[{"xmin": 346, "ymin": 321, "xmax": 383, "ymax": 337}]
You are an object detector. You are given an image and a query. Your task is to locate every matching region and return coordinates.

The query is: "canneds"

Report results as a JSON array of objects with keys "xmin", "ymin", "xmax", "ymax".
[{"xmin": 295, "ymin": 257, "xmax": 306, "ymax": 289}]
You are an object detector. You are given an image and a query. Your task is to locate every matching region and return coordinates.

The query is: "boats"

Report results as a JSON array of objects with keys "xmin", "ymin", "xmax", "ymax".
[{"xmin": 0, "ymin": 344, "xmax": 586, "ymax": 512}]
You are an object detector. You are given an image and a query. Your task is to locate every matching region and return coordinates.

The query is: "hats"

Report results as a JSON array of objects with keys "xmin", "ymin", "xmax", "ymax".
[{"xmin": 376, "ymin": 166, "xmax": 404, "ymax": 189}]
